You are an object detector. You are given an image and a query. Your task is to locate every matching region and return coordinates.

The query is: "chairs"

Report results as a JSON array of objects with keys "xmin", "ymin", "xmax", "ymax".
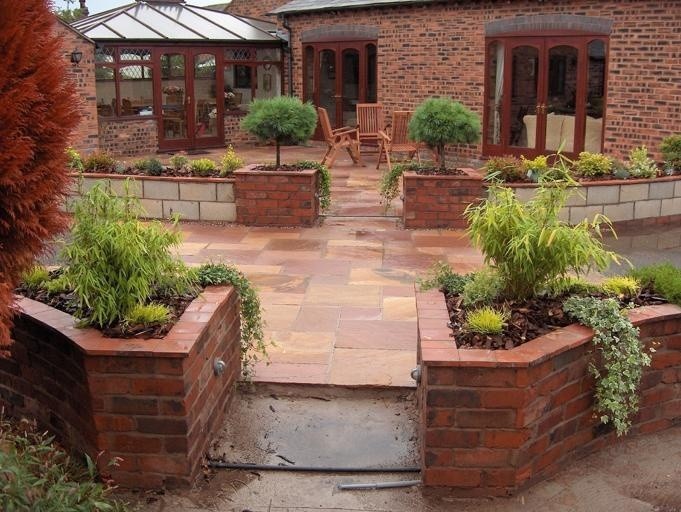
[
  {"xmin": 509, "ymin": 104, "xmax": 603, "ymax": 155},
  {"xmin": 355, "ymin": 102, "xmax": 387, "ymax": 160},
  {"xmin": 376, "ymin": 110, "xmax": 421, "ymax": 173},
  {"xmin": 318, "ymin": 107, "xmax": 366, "ymax": 168},
  {"xmin": 96, "ymin": 92, "xmax": 244, "ymax": 138}
]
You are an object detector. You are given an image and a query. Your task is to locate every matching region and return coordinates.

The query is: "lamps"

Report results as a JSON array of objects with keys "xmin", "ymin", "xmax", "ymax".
[{"xmin": 71, "ymin": 47, "xmax": 82, "ymax": 72}]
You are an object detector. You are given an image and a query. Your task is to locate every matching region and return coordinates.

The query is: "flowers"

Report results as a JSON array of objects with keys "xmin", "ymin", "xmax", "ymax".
[{"xmin": 163, "ymin": 85, "xmax": 184, "ymax": 95}]
